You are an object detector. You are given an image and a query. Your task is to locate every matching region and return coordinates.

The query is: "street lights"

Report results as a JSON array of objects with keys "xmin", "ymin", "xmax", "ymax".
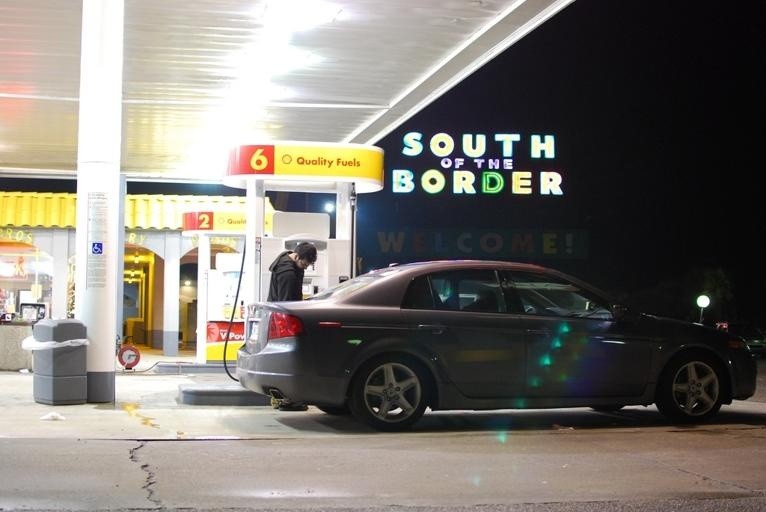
[{"xmin": 696, "ymin": 293, "xmax": 711, "ymax": 324}]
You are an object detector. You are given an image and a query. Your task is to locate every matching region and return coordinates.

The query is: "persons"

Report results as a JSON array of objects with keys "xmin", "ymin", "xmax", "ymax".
[{"xmin": 267, "ymin": 242, "xmax": 317, "ymax": 302}]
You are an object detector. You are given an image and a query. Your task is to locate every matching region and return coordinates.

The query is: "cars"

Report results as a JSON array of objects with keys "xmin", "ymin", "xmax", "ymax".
[
  {"xmin": 747, "ymin": 333, "xmax": 766, "ymax": 357},
  {"xmin": 236, "ymin": 257, "xmax": 757, "ymax": 432}
]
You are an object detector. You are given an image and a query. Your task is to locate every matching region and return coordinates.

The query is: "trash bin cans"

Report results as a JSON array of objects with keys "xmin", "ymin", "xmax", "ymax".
[{"xmin": 33, "ymin": 320, "xmax": 87, "ymax": 406}]
